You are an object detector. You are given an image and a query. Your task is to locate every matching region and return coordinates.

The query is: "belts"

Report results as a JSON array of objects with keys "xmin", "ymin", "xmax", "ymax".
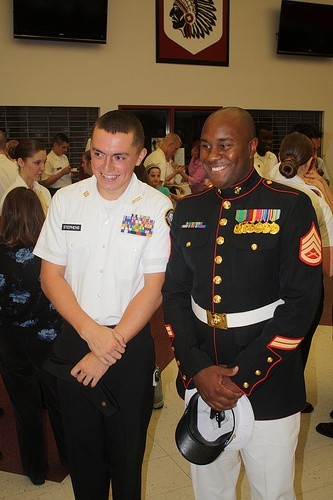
[{"xmin": 191, "ymin": 295, "xmax": 285, "ymax": 329}]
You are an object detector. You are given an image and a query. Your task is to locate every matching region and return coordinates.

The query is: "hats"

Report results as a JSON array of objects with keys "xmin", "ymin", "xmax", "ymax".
[{"xmin": 174, "ymin": 388, "xmax": 255, "ymax": 466}]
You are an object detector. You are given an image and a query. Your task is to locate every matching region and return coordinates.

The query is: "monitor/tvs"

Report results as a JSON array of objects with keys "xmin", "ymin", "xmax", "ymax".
[
  {"xmin": 11, "ymin": 0, "xmax": 108, "ymax": 44},
  {"xmin": 276, "ymin": 0, "xmax": 333, "ymax": 58}
]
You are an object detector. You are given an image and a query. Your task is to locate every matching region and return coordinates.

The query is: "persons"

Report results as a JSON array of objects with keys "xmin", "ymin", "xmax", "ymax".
[{"xmin": 0, "ymin": 107, "xmax": 333, "ymax": 500}]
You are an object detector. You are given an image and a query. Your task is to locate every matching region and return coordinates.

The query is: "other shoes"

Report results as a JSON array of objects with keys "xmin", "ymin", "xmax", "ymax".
[
  {"xmin": 300, "ymin": 401, "xmax": 314, "ymax": 413},
  {"xmin": 316, "ymin": 422, "xmax": 333, "ymax": 438}
]
